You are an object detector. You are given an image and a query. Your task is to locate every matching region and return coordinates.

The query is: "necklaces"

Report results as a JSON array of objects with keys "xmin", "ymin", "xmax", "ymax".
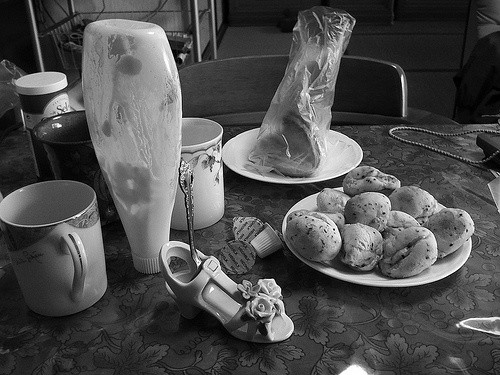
[{"xmin": 388, "ymin": 126, "xmax": 500, "ymax": 164}]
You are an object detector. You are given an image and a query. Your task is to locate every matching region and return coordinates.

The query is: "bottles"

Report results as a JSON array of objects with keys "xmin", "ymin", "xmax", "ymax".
[
  {"xmin": 14, "ymin": 71, "xmax": 70, "ymax": 179},
  {"xmin": 81, "ymin": 20, "xmax": 183, "ymax": 273}
]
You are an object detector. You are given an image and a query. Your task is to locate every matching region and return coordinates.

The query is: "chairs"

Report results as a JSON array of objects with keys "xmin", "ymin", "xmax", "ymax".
[
  {"xmin": 179, "ymin": 54, "xmax": 408, "ymax": 125},
  {"xmin": 452, "ymin": 0, "xmax": 500, "ymax": 124}
]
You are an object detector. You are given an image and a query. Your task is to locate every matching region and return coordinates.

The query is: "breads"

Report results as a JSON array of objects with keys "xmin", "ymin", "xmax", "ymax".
[
  {"xmin": 284, "ymin": 165, "xmax": 476, "ymax": 277},
  {"xmin": 251, "ymin": 112, "xmax": 323, "ymax": 177}
]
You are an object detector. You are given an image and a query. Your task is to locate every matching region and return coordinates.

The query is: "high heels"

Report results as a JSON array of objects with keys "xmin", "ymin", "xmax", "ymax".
[{"xmin": 157, "ymin": 238, "xmax": 298, "ymax": 347}]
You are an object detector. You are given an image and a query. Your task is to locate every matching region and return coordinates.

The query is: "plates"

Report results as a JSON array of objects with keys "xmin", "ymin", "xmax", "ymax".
[
  {"xmin": 281, "ymin": 187, "xmax": 472, "ymax": 287},
  {"xmin": 222, "ymin": 127, "xmax": 363, "ymax": 184}
]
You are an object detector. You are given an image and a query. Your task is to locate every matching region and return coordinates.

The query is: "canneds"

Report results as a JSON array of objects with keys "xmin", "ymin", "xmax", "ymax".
[{"xmin": 15, "ymin": 72, "xmax": 72, "ymax": 177}]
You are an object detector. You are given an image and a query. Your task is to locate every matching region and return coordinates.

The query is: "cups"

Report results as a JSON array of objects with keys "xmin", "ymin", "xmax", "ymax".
[
  {"xmin": 170, "ymin": 119, "xmax": 225, "ymax": 230},
  {"xmin": 0, "ymin": 181, "xmax": 108, "ymax": 316},
  {"xmin": 33, "ymin": 110, "xmax": 117, "ymax": 220}
]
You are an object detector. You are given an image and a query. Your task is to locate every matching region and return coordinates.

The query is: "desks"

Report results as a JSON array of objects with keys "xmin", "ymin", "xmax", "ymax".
[{"xmin": 0, "ymin": 124, "xmax": 500, "ymax": 375}]
